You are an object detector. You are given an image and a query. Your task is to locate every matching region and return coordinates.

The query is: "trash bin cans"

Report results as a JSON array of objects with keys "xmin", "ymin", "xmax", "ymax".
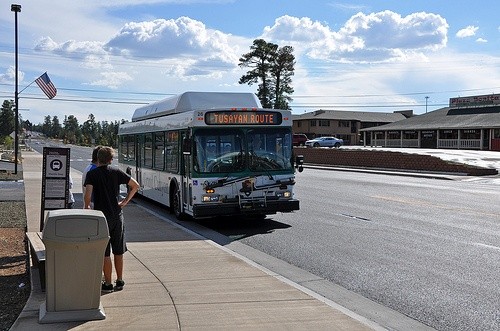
[{"xmin": 38, "ymin": 209, "xmax": 111, "ymax": 324}]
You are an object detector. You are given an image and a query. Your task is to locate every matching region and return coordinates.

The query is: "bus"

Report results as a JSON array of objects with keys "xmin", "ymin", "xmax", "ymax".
[{"xmin": 117, "ymin": 90, "xmax": 305, "ymax": 223}]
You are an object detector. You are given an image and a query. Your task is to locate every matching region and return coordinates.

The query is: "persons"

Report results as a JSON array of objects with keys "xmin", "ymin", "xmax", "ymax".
[
  {"xmin": 68, "ymin": 146, "xmax": 140, "ymax": 292},
  {"xmin": 251, "ymin": 138, "xmax": 269, "ymax": 167}
]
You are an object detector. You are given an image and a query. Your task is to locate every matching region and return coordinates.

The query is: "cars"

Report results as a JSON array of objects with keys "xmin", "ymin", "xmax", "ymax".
[{"xmin": 305, "ymin": 135, "xmax": 344, "ymax": 148}]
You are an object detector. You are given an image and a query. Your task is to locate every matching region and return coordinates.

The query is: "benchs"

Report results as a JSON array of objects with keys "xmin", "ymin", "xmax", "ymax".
[{"xmin": 26, "ymin": 231, "xmax": 46, "ymax": 292}]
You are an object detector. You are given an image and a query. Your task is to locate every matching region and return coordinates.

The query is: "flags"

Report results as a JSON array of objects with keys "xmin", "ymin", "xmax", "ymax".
[{"xmin": 35, "ymin": 72, "xmax": 57, "ymax": 99}]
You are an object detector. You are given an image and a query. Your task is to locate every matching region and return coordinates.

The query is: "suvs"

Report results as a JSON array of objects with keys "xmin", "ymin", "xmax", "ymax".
[{"xmin": 277, "ymin": 133, "xmax": 309, "ymax": 148}]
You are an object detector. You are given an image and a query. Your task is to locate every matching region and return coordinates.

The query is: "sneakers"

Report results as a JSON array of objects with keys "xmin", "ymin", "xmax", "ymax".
[
  {"xmin": 114, "ymin": 280, "xmax": 126, "ymax": 290},
  {"xmin": 101, "ymin": 282, "xmax": 115, "ymax": 293}
]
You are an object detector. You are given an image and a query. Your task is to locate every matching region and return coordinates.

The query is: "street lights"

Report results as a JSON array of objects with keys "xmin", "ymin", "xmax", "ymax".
[
  {"xmin": 425, "ymin": 96, "xmax": 430, "ymax": 114},
  {"xmin": 11, "ymin": 3, "xmax": 22, "ymax": 175}
]
